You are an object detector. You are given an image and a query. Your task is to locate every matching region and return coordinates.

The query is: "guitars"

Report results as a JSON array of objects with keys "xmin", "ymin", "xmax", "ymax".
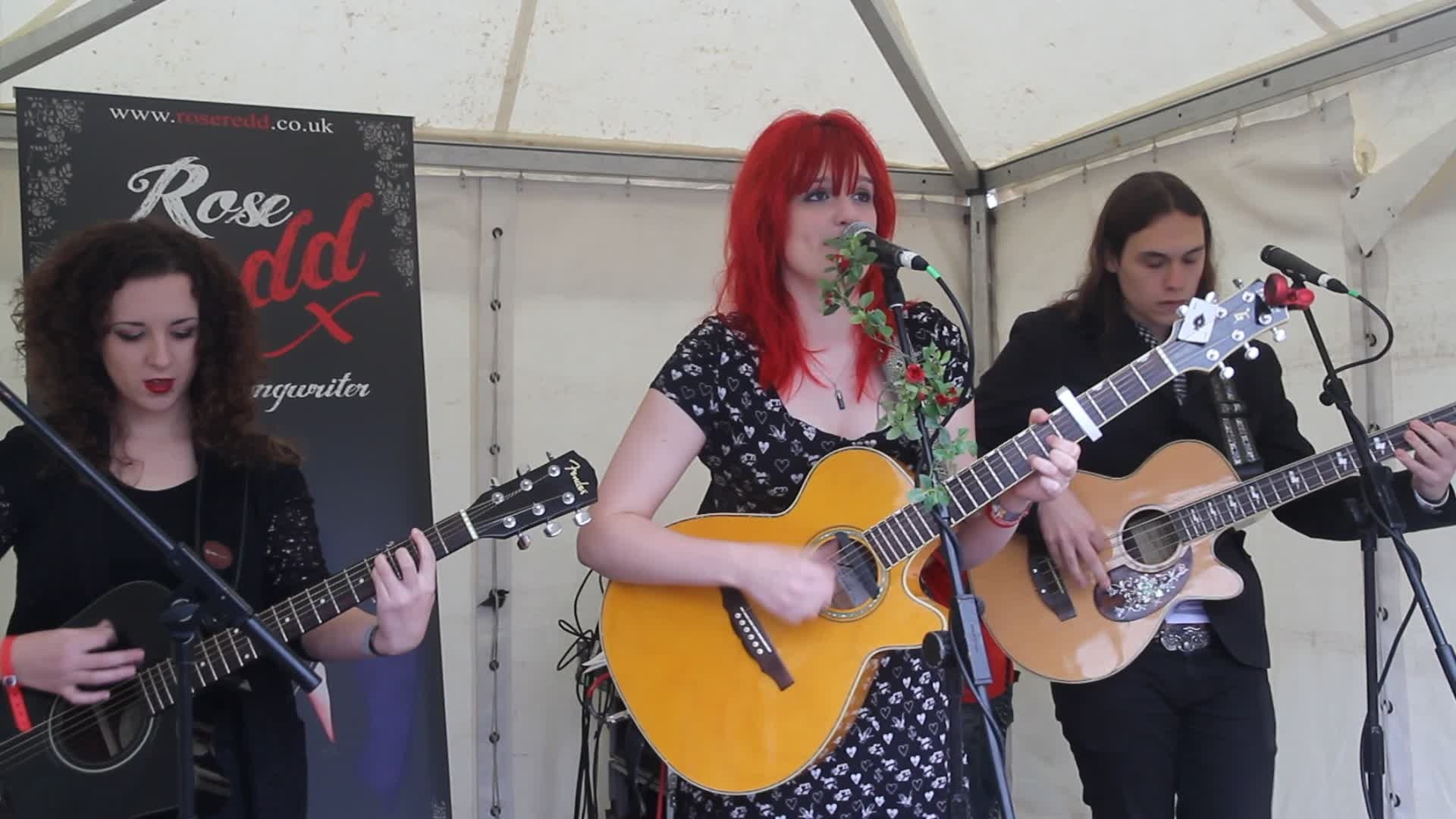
[
  {"xmin": 599, "ymin": 277, "xmax": 1291, "ymax": 797},
  {"xmin": 964, "ymin": 399, "xmax": 1456, "ymax": 685},
  {"xmin": 0, "ymin": 447, "xmax": 602, "ymax": 819}
]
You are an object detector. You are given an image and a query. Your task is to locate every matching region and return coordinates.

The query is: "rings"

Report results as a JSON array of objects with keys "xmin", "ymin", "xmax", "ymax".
[{"xmin": 1062, "ymin": 477, "xmax": 1070, "ymax": 488}]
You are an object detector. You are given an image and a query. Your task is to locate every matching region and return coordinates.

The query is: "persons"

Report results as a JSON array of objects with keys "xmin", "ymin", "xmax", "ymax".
[
  {"xmin": 974, "ymin": 172, "xmax": 1456, "ymax": 819},
  {"xmin": 0, "ymin": 219, "xmax": 436, "ymax": 819},
  {"xmin": 577, "ymin": 109, "xmax": 1081, "ymax": 819}
]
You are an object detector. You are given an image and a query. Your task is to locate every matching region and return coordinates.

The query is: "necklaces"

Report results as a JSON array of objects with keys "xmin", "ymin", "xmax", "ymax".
[{"xmin": 807, "ymin": 350, "xmax": 857, "ymax": 409}]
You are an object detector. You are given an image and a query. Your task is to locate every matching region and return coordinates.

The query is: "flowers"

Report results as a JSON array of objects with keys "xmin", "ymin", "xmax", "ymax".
[{"xmin": 825, "ymin": 234, "xmax": 979, "ymax": 512}]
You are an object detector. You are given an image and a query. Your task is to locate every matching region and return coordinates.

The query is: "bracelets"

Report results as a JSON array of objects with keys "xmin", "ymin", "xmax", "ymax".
[
  {"xmin": 368, "ymin": 625, "xmax": 388, "ymax": 656},
  {"xmin": 0, "ymin": 636, "xmax": 30, "ymax": 733},
  {"xmin": 986, "ymin": 500, "xmax": 1032, "ymax": 528}
]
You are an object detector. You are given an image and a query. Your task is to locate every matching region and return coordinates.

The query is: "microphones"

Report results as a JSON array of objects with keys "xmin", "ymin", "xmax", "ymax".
[
  {"xmin": 1260, "ymin": 245, "xmax": 1349, "ymax": 294},
  {"xmin": 845, "ymin": 221, "xmax": 928, "ymax": 271}
]
